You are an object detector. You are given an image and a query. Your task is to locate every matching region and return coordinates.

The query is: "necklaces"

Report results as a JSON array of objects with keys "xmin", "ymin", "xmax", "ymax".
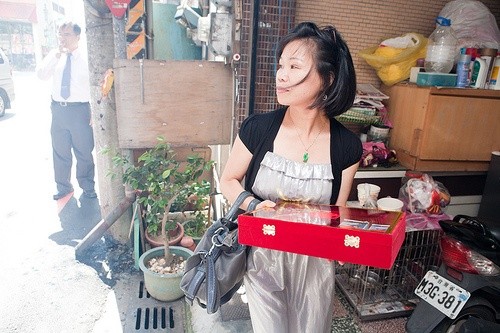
[{"xmin": 290, "ymin": 118, "xmax": 326, "ymax": 162}]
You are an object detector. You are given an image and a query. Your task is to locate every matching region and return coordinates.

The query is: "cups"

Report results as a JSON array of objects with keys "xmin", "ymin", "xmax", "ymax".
[
  {"xmin": 377, "ymin": 197, "xmax": 404, "ymax": 211},
  {"xmin": 357, "ymin": 183, "xmax": 381, "ymax": 206},
  {"xmin": 455, "ymin": 55, "xmax": 471, "ymax": 88}
]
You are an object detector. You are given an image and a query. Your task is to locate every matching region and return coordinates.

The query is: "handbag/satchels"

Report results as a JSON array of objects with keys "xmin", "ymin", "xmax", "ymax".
[{"xmin": 179, "ymin": 188, "xmax": 261, "ymax": 314}]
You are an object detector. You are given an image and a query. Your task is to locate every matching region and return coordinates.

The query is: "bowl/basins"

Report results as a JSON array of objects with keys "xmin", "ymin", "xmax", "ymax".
[{"xmin": 349, "ymin": 269, "xmax": 381, "ymax": 288}]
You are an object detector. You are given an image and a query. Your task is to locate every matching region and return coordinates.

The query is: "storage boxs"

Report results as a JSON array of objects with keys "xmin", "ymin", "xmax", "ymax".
[
  {"xmin": 237, "ymin": 201, "xmax": 406, "ymax": 270},
  {"xmin": 409, "ymin": 67, "xmax": 457, "ymax": 86}
]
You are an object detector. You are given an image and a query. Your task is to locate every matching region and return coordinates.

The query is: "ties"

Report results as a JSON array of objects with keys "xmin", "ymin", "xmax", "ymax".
[{"xmin": 61, "ymin": 51, "xmax": 73, "ymax": 100}]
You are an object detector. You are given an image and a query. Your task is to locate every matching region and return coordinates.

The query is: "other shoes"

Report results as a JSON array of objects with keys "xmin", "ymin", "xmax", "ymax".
[
  {"xmin": 53, "ymin": 189, "xmax": 74, "ymax": 200},
  {"xmin": 84, "ymin": 189, "xmax": 97, "ymax": 198}
]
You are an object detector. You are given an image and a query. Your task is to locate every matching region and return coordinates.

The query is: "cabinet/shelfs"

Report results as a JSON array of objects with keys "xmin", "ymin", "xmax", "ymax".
[{"xmin": 379, "ymin": 82, "xmax": 500, "ymax": 171}]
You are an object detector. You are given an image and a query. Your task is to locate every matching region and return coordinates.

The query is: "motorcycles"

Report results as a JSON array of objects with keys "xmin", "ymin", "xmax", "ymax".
[{"xmin": 403, "ymin": 215, "xmax": 500, "ymax": 333}]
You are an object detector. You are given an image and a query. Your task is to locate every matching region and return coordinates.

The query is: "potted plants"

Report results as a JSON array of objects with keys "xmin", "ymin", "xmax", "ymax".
[{"xmin": 97, "ymin": 136, "xmax": 217, "ymax": 301}]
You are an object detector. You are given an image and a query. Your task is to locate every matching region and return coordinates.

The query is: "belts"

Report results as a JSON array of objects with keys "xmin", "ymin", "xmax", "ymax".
[{"xmin": 53, "ymin": 101, "xmax": 81, "ymax": 107}]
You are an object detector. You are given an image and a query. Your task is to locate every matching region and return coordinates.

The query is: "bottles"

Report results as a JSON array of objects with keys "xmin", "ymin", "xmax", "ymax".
[{"xmin": 424, "ymin": 17, "xmax": 458, "ymax": 75}]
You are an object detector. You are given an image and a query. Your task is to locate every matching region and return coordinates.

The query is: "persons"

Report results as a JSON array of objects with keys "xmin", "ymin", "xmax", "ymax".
[
  {"xmin": 219, "ymin": 23, "xmax": 364, "ymax": 333},
  {"xmin": 36, "ymin": 22, "xmax": 97, "ymax": 200}
]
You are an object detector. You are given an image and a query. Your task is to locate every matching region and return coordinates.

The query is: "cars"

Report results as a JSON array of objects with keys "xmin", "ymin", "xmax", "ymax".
[{"xmin": 0, "ymin": 50, "xmax": 16, "ymax": 119}]
